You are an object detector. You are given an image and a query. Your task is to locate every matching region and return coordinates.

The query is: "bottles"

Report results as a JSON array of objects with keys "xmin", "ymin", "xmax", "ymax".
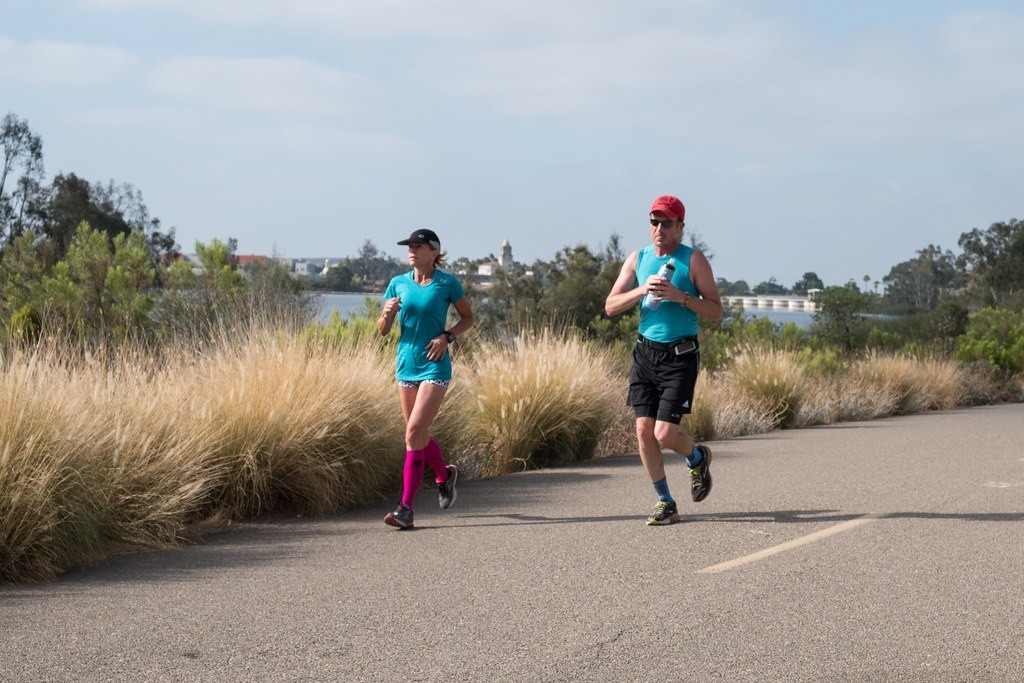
[{"xmin": 642, "ymin": 258, "xmax": 676, "ymax": 312}]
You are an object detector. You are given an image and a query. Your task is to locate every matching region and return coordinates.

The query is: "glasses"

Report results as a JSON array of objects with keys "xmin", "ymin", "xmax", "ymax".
[{"xmin": 650, "ymin": 218, "xmax": 678, "ymax": 229}]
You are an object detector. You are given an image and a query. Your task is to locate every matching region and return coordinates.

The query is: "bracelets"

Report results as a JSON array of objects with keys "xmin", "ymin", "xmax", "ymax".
[{"xmin": 681, "ymin": 291, "xmax": 690, "ymax": 307}]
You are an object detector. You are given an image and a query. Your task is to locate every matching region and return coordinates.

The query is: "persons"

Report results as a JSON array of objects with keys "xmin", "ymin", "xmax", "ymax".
[
  {"xmin": 378, "ymin": 229, "xmax": 474, "ymax": 529},
  {"xmin": 605, "ymin": 196, "xmax": 723, "ymax": 525}
]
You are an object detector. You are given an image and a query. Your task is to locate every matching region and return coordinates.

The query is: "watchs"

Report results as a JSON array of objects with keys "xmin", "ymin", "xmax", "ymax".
[{"xmin": 442, "ymin": 331, "xmax": 455, "ymax": 343}]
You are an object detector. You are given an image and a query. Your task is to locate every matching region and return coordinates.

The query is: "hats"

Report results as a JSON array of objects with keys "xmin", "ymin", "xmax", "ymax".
[
  {"xmin": 649, "ymin": 195, "xmax": 685, "ymax": 222},
  {"xmin": 396, "ymin": 229, "xmax": 440, "ymax": 250}
]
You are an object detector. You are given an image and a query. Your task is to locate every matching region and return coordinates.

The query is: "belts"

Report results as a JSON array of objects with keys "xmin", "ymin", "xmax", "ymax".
[{"xmin": 638, "ymin": 334, "xmax": 696, "ymax": 351}]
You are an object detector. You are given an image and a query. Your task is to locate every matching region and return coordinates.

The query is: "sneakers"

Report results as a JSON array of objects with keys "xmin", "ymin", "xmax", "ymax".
[
  {"xmin": 646, "ymin": 499, "xmax": 681, "ymax": 525},
  {"xmin": 384, "ymin": 494, "xmax": 414, "ymax": 529},
  {"xmin": 435, "ymin": 464, "xmax": 458, "ymax": 511},
  {"xmin": 687, "ymin": 445, "xmax": 713, "ymax": 503}
]
